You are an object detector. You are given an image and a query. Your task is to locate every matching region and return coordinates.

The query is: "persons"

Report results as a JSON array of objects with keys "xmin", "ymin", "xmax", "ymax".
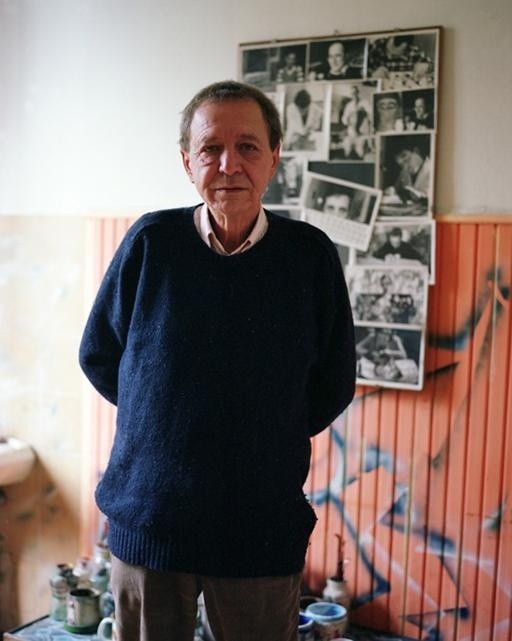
[{"xmin": 80, "ymin": 81, "xmax": 359, "ymax": 640}]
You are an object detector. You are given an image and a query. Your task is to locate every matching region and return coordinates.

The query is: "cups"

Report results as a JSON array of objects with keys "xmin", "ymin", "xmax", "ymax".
[
  {"xmin": 305, "ymin": 602, "xmax": 349, "ymax": 641},
  {"xmin": 64, "ymin": 589, "xmax": 103, "ymax": 636}
]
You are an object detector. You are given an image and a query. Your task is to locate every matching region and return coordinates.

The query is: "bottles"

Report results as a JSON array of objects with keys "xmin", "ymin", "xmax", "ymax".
[{"xmin": 49, "ymin": 564, "xmax": 80, "ymax": 623}]
